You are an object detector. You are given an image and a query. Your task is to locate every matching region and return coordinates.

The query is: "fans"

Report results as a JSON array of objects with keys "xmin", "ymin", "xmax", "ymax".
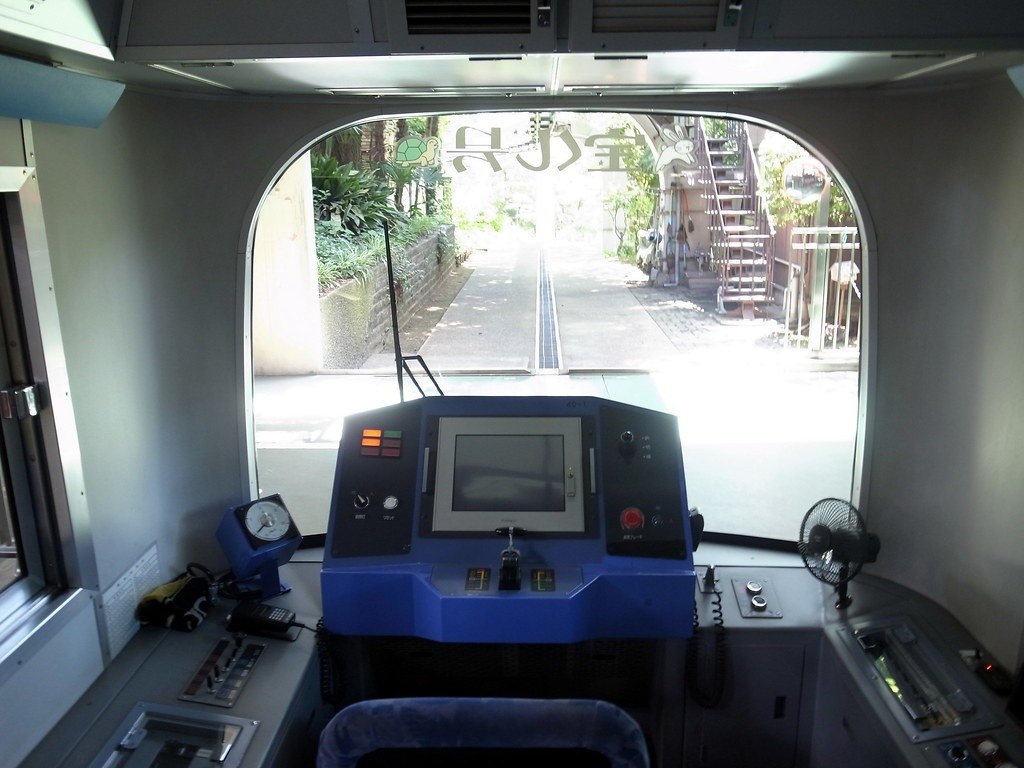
[{"xmin": 799, "ymin": 496, "xmax": 884, "ymax": 606}]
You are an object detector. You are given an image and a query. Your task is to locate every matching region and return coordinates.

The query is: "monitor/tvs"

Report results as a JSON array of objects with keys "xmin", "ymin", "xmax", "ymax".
[{"xmin": 432, "ymin": 416, "xmax": 584, "ymax": 532}]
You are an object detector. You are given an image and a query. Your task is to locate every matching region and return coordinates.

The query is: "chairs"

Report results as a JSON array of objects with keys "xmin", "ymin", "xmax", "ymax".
[{"xmin": 318, "ymin": 698, "xmax": 651, "ymax": 768}]
